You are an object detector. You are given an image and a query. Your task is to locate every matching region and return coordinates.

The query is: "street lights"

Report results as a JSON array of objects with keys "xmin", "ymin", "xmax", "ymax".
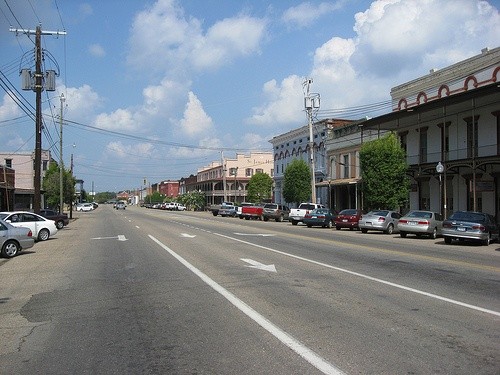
[
  {"xmin": 33, "ymin": 93, "xmax": 65, "ymax": 214},
  {"xmin": 436, "ymin": 162, "xmax": 444, "ymax": 213},
  {"xmin": 59, "ymin": 143, "xmax": 76, "ymax": 215}
]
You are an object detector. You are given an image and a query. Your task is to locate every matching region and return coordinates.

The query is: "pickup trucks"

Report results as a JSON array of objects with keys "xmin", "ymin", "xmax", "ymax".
[
  {"xmin": 209, "ymin": 201, "xmax": 237, "ymax": 216},
  {"xmin": 289, "ymin": 202, "xmax": 326, "ymax": 226},
  {"xmin": 241, "ymin": 203, "xmax": 268, "ymax": 220}
]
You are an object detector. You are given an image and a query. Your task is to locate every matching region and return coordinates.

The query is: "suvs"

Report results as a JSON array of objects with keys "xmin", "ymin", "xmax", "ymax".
[{"xmin": 263, "ymin": 202, "xmax": 290, "ymax": 222}]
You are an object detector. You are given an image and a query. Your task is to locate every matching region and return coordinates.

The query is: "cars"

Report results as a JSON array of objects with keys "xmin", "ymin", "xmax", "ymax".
[
  {"xmin": 442, "ymin": 209, "xmax": 500, "ymax": 246},
  {"xmin": 113, "ymin": 201, "xmax": 127, "ymax": 211},
  {"xmin": 335, "ymin": 209, "xmax": 364, "ymax": 230},
  {"xmin": 30, "ymin": 210, "xmax": 70, "ymax": 229},
  {"xmin": 0, "ymin": 211, "xmax": 58, "ymax": 241},
  {"xmin": 358, "ymin": 210, "xmax": 403, "ymax": 234},
  {"xmin": 396, "ymin": 210, "xmax": 444, "ymax": 239},
  {"xmin": 304, "ymin": 208, "xmax": 339, "ymax": 229},
  {"xmin": 140, "ymin": 201, "xmax": 186, "ymax": 211},
  {"xmin": 73, "ymin": 202, "xmax": 97, "ymax": 212},
  {"xmin": 218, "ymin": 204, "xmax": 243, "ymax": 219},
  {"xmin": 0, "ymin": 218, "xmax": 35, "ymax": 259}
]
